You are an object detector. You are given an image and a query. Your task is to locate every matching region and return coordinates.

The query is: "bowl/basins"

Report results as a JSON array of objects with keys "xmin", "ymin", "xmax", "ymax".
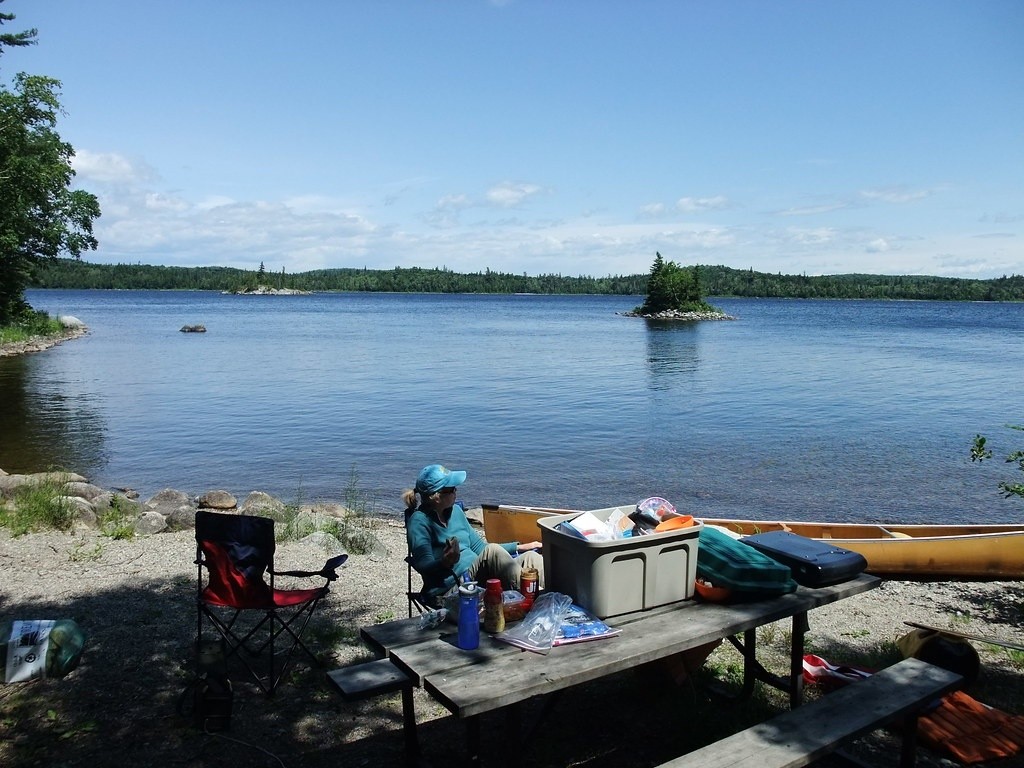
[{"xmin": 695, "ymin": 577, "xmax": 732, "ymax": 603}]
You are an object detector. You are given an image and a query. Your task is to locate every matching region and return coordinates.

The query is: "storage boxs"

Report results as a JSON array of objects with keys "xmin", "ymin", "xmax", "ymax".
[
  {"xmin": 698, "ymin": 527, "xmax": 798, "ymax": 595},
  {"xmin": 535, "ymin": 504, "xmax": 706, "ymax": 621},
  {"xmin": 738, "ymin": 529, "xmax": 870, "ymax": 587}
]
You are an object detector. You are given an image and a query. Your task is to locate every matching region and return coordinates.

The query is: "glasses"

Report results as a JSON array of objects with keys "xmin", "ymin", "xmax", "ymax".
[{"xmin": 443, "ymin": 487, "xmax": 457, "ymax": 494}]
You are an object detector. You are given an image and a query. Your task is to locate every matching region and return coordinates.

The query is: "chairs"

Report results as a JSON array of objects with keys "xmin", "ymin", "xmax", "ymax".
[
  {"xmin": 404, "ymin": 508, "xmax": 443, "ymax": 619},
  {"xmin": 192, "ymin": 511, "xmax": 349, "ymax": 701}
]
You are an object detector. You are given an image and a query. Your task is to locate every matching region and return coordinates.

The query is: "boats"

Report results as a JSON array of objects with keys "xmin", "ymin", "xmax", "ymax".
[{"xmin": 484, "ymin": 503, "xmax": 1024, "ymax": 578}]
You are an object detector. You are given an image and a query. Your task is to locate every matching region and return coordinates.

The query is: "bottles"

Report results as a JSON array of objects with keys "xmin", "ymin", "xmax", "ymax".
[
  {"xmin": 0, "ymin": 619, "xmax": 86, "ymax": 677},
  {"xmin": 483, "ymin": 579, "xmax": 504, "ymax": 633}
]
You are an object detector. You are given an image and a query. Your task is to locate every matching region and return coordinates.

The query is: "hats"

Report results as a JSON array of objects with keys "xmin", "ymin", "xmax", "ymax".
[{"xmin": 416, "ymin": 464, "xmax": 466, "ymax": 498}]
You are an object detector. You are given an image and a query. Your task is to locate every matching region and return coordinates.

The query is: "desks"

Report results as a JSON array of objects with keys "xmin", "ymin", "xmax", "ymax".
[{"xmin": 357, "ymin": 560, "xmax": 881, "ymax": 768}]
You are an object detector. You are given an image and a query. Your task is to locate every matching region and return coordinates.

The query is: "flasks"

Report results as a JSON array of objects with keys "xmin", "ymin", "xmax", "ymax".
[{"xmin": 456, "ymin": 580, "xmax": 481, "ymax": 650}]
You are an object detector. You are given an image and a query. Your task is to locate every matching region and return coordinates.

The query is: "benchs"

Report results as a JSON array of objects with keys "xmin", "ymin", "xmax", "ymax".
[
  {"xmin": 325, "ymin": 656, "xmax": 424, "ymax": 766},
  {"xmin": 653, "ymin": 655, "xmax": 961, "ymax": 768}
]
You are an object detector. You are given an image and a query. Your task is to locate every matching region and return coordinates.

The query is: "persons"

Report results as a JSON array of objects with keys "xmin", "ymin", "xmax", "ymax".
[{"xmin": 400, "ymin": 464, "xmax": 546, "ymax": 609}]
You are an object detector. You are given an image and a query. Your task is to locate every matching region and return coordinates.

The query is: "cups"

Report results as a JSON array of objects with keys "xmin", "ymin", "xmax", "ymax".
[{"xmin": 520, "ymin": 568, "xmax": 540, "ymax": 602}]
